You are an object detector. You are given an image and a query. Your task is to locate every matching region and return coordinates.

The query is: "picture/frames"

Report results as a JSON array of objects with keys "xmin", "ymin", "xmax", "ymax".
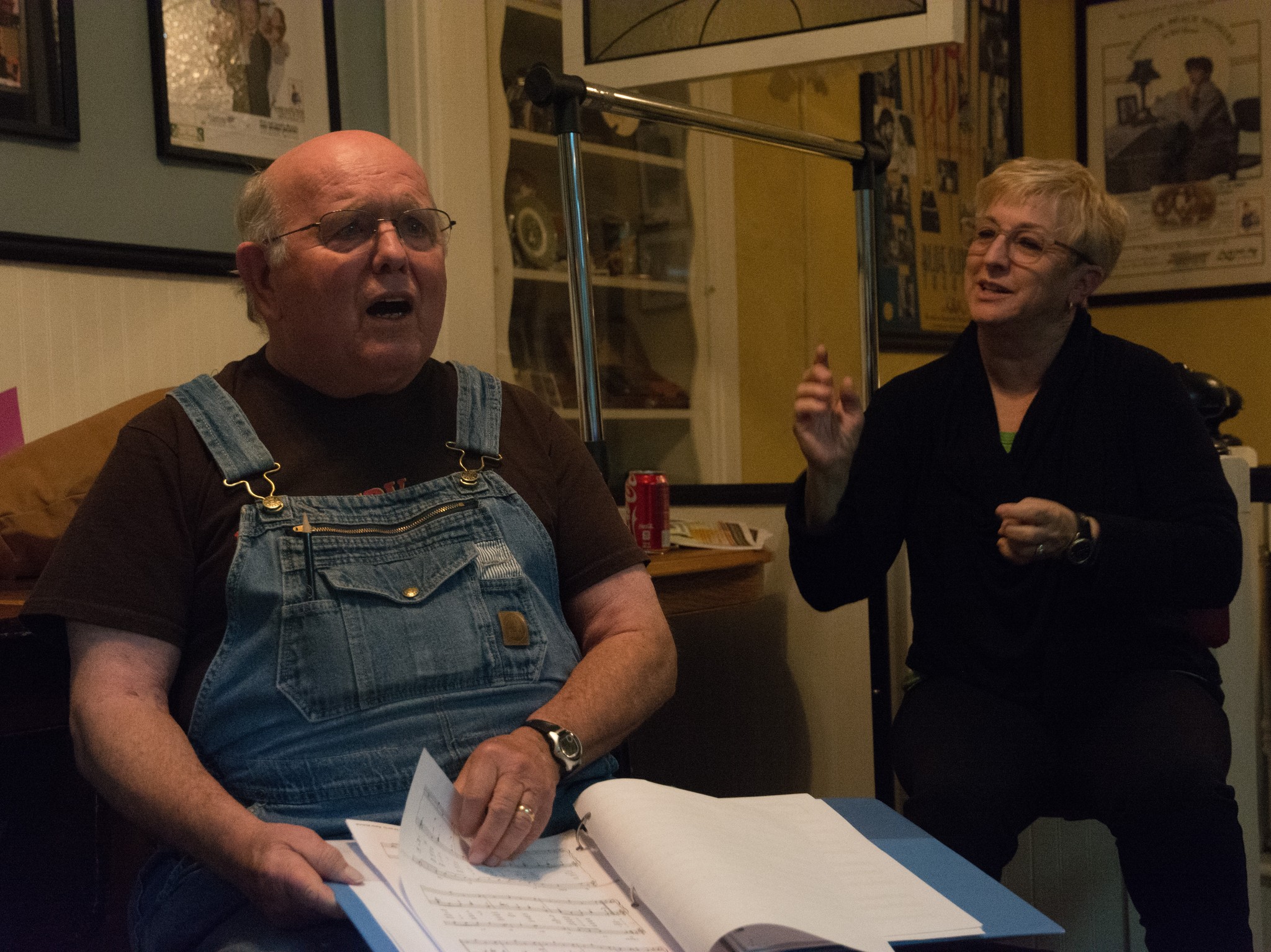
[
  {"xmin": 0, "ymin": 0, "xmax": 80, "ymax": 156},
  {"xmin": 1073, "ymin": 0, "xmax": 1271, "ymax": 311},
  {"xmin": 147, "ymin": 0, "xmax": 342, "ymax": 176}
]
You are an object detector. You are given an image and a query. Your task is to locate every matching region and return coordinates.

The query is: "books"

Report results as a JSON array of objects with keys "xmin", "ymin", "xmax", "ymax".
[{"xmin": 329, "ymin": 746, "xmax": 1067, "ymax": 952}]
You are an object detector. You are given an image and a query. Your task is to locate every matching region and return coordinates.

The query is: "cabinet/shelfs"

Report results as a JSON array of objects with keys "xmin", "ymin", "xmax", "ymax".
[{"xmin": 493, "ymin": 0, "xmax": 747, "ymax": 492}]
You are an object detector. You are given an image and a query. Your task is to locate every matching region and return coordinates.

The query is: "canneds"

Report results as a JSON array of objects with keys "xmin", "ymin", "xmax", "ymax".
[{"xmin": 625, "ymin": 470, "xmax": 671, "ymax": 554}]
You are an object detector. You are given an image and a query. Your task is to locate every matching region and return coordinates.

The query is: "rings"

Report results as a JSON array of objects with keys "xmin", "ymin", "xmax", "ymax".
[
  {"xmin": 1032, "ymin": 544, "xmax": 1045, "ymax": 561},
  {"xmin": 518, "ymin": 802, "xmax": 538, "ymax": 823}
]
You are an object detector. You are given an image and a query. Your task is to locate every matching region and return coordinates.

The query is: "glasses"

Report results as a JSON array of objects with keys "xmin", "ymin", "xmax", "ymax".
[
  {"xmin": 262, "ymin": 208, "xmax": 458, "ymax": 252},
  {"xmin": 961, "ymin": 214, "xmax": 1104, "ymax": 268}
]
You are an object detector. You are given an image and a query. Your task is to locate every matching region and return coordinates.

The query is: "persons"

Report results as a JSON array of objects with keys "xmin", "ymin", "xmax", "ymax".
[
  {"xmin": 1178, "ymin": 56, "xmax": 1238, "ymax": 181},
  {"xmin": 783, "ymin": 158, "xmax": 1252, "ymax": 952},
  {"xmin": 240, "ymin": 0, "xmax": 290, "ymax": 117},
  {"xmin": 23, "ymin": 130, "xmax": 662, "ymax": 952}
]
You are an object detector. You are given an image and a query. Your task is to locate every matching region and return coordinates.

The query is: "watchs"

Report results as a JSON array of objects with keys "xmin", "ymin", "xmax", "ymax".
[
  {"xmin": 1065, "ymin": 511, "xmax": 1096, "ymax": 568},
  {"xmin": 518, "ymin": 719, "xmax": 583, "ymax": 775}
]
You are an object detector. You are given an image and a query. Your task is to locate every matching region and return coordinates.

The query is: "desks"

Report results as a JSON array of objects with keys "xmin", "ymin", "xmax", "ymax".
[{"xmin": 605, "ymin": 547, "xmax": 782, "ymax": 796}]
